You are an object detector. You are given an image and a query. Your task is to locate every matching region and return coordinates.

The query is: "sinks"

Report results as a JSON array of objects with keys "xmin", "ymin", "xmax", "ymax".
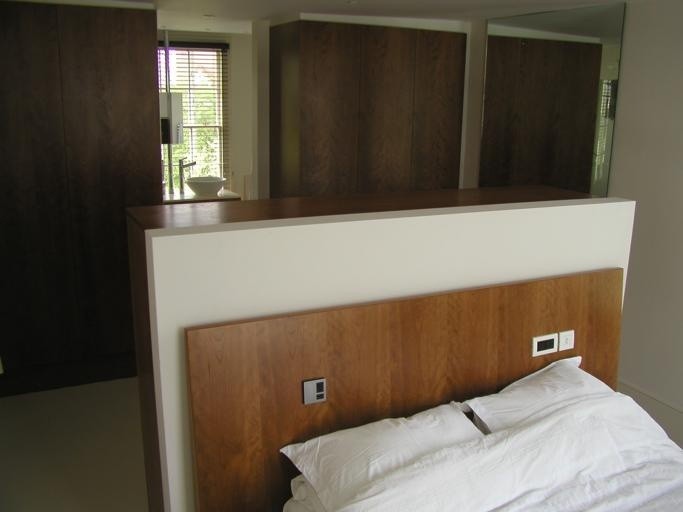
[{"xmin": 185, "ymin": 177, "xmax": 227, "ymax": 196}]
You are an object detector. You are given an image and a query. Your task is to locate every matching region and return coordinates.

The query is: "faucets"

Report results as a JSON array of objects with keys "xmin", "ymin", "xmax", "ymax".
[{"xmin": 178, "ymin": 157, "xmax": 198, "ymax": 194}]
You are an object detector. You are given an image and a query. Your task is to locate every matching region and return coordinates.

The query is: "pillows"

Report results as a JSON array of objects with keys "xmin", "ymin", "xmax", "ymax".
[
  {"xmin": 278, "ymin": 401, "xmax": 485, "ymax": 510},
  {"xmin": 454, "ymin": 356, "xmax": 618, "ymax": 433}
]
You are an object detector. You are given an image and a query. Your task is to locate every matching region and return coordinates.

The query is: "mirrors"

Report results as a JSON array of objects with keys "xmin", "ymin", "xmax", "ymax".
[{"xmin": 478, "ymin": 3, "xmax": 628, "ymax": 198}]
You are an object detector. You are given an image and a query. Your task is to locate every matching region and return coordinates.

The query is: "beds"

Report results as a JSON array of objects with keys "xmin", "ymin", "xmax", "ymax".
[{"xmin": 185, "ymin": 268, "xmax": 683, "ymax": 512}]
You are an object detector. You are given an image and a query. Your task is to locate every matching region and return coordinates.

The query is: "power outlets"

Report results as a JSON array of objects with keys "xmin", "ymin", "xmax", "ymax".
[{"xmin": 557, "ymin": 330, "xmax": 575, "ymax": 351}]
